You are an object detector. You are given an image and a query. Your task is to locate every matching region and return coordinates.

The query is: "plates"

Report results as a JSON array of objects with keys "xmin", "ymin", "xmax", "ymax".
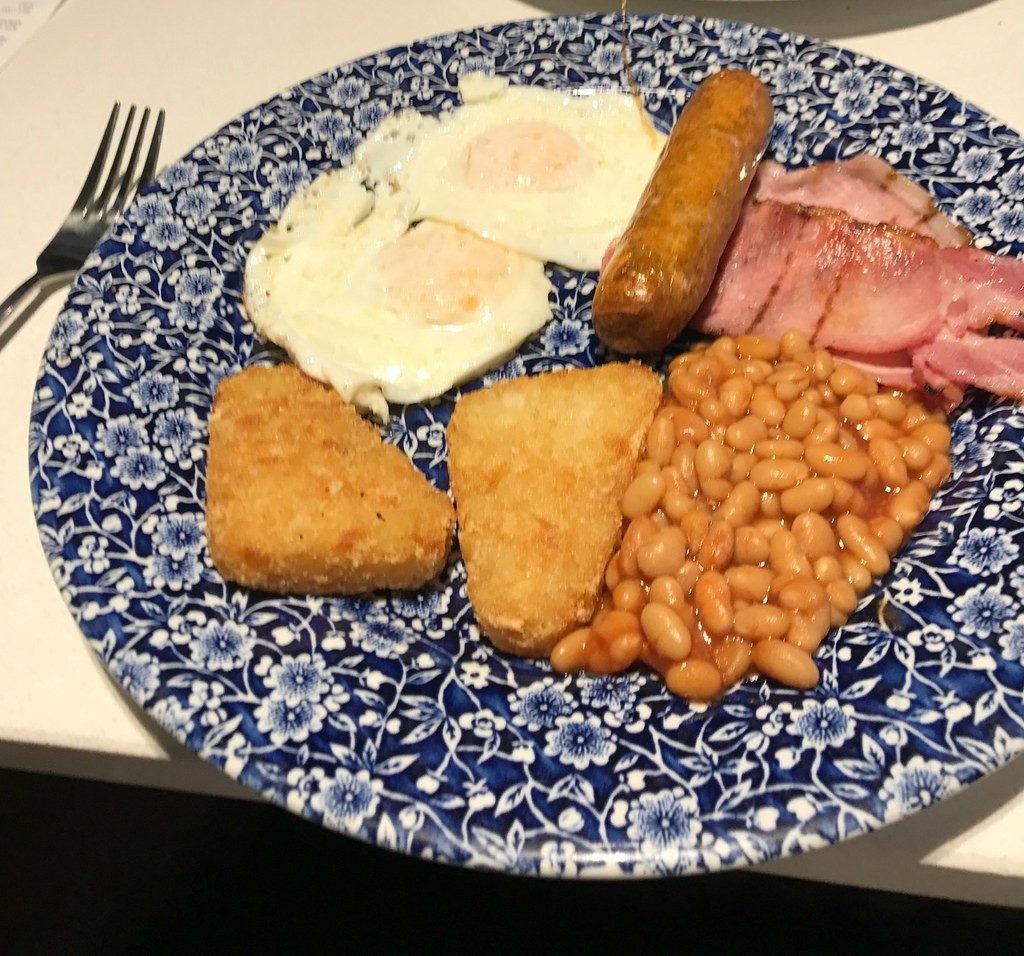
[{"xmin": 26, "ymin": 15, "xmax": 1024, "ymax": 882}]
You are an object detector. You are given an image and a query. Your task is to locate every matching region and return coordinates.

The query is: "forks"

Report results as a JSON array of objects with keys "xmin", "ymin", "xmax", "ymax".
[{"xmin": 0, "ymin": 101, "xmax": 166, "ymax": 351}]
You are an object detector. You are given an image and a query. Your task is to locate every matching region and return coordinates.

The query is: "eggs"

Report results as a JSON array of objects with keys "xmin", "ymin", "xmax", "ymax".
[
  {"xmin": 353, "ymin": 69, "xmax": 674, "ymax": 273},
  {"xmin": 244, "ymin": 163, "xmax": 554, "ymax": 422}
]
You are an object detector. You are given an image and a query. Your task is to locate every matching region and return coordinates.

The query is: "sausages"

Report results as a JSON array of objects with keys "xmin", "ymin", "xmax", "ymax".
[{"xmin": 591, "ymin": 68, "xmax": 774, "ymax": 353}]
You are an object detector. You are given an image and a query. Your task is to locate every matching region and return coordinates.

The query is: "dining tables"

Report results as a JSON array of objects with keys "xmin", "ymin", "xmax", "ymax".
[{"xmin": 0, "ymin": 0, "xmax": 1024, "ymax": 911}]
